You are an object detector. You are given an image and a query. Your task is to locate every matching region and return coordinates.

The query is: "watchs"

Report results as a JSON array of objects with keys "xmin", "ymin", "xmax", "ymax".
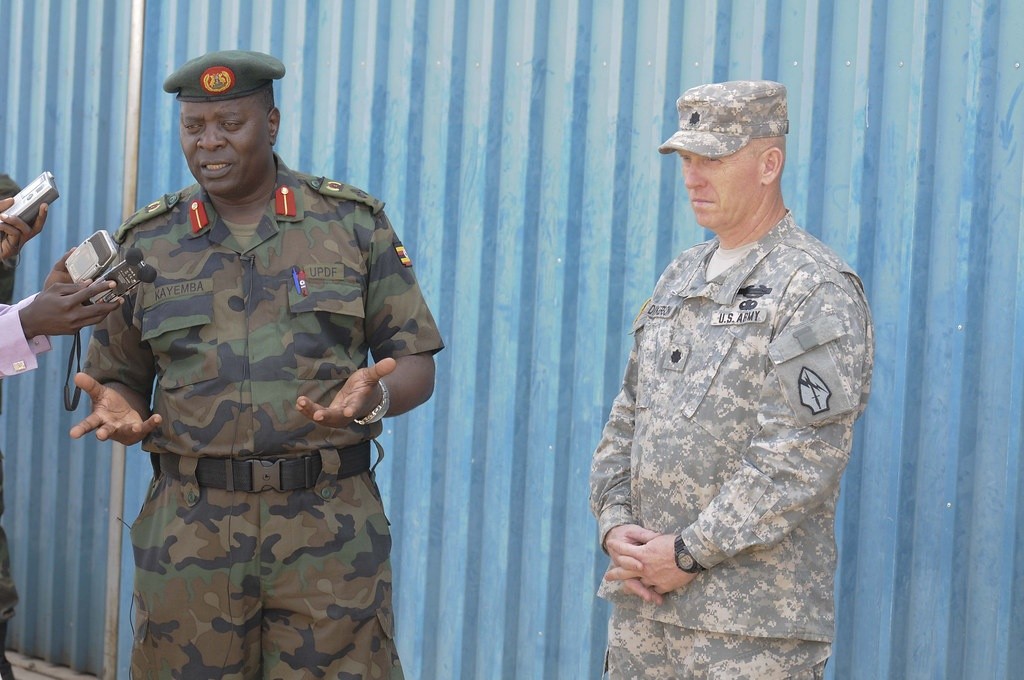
[
  {"xmin": 674, "ymin": 535, "xmax": 704, "ymax": 574},
  {"xmin": 354, "ymin": 379, "xmax": 390, "ymax": 425}
]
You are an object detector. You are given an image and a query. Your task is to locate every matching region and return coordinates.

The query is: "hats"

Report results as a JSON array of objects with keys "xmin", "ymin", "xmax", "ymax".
[
  {"xmin": 163, "ymin": 51, "xmax": 286, "ymax": 103},
  {"xmin": 658, "ymin": 81, "xmax": 789, "ymax": 158}
]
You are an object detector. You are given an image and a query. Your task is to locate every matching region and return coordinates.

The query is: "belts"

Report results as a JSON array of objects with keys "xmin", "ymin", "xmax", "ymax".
[{"xmin": 158, "ymin": 440, "xmax": 371, "ymax": 493}]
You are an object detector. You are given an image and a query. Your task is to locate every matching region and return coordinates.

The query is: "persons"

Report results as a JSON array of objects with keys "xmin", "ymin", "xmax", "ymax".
[
  {"xmin": 0, "ymin": 175, "xmax": 124, "ymax": 680},
  {"xmin": 69, "ymin": 52, "xmax": 444, "ymax": 679},
  {"xmin": 590, "ymin": 82, "xmax": 871, "ymax": 680}
]
